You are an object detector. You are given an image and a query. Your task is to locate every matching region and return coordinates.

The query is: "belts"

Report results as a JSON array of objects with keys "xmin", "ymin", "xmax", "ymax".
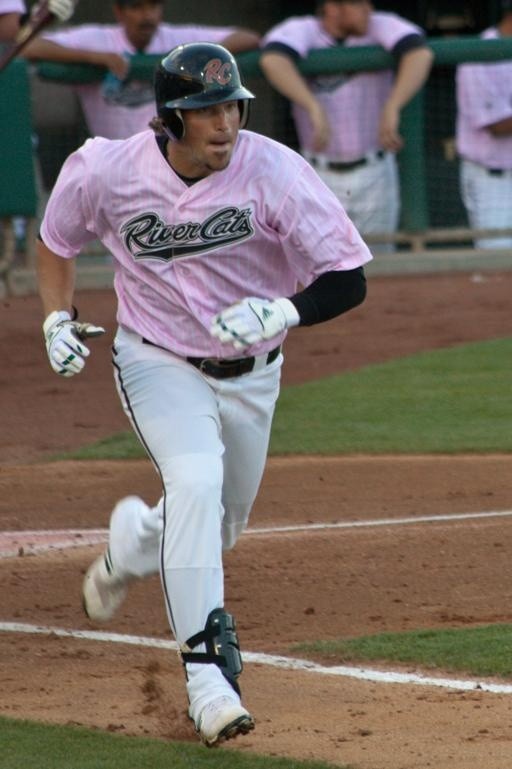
[
  {"xmin": 142, "ymin": 337, "xmax": 281, "ymax": 381},
  {"xmin": 308, "ymin": 150, "xmax": 388, "ymax": 174},
  {"xmin": 486, "ymin": 167, "xmax": 507, "ymax": 180}
]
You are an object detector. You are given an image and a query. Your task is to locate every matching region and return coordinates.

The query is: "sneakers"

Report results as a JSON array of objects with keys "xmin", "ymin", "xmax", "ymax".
[
  {"xmin": 183, "ymin": 662, "xmax": 256, "ymax": 750},
  {"xmin": 79, "ymin": 494, "xmax": 149, "ymax": 621}
]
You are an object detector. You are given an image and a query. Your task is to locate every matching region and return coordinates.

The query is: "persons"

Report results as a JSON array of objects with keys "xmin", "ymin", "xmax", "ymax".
[
  {"xmin": 453, "ymin": 0, "xmax": 512, "ymax": 251},
  {"xmin": 255, "ymin": 1, "xmax": 435, "ymax": 255},
  {"xmin": 34, "ymin": 43, "xmax": 373, "ymax": 746},
  {"xmin": 24, "ymin": 1, "xmax": 261, "ymax": 139},
  {"xmin": 0, "ymin": 1, "xmax": 27, "ymax": 68}
]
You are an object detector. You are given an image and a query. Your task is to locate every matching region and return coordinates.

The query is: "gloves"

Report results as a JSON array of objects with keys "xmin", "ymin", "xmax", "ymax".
[
  {"xmin": 41, "ymin": 309, "xmax": 106, "ymax": 379},
  {"xmin": 209, "ymin": 296, "xmax": 302, "ymax": 352},
  {"xmin": 28, "ymin": 0, "xmax": 75, "ymax": 23}
]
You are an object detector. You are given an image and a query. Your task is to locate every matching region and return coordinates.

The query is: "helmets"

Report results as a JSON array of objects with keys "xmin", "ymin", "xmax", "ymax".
[{"xmin": 152, "ymin": 41, "xmax": 257, "ymax": 143}]
